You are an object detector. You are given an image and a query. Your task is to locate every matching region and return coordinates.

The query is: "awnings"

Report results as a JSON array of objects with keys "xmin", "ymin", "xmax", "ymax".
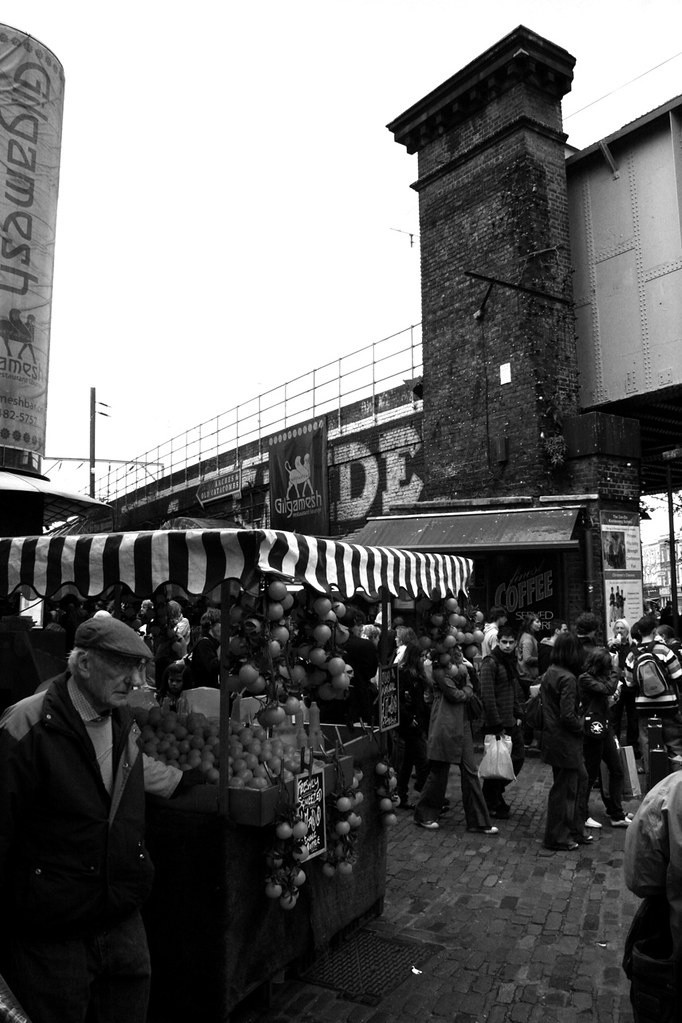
[{"xmin": 352, "ymin": 505, "xmax": 589, "ymax": 549}]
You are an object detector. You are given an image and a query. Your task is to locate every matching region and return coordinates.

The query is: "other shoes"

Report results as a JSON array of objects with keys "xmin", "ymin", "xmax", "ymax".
[
  {"xmin": 585, "ymin": 836, "xmax": 593, "ymax": 843},
  {"xmin": 593, "ymin": 779, "xmax": 603, "ymax": 789},
  {"xmin": 416, "ymin": 819, "xmax": 439, "ymax": 829},
  {"xmin": 574, "ymin": 843, "xmax": 579, "ymax": 848},
  {"xmin": 411, "ymin": 789, "xmax": 422, "ymax": 799},
  {"xmin": 610, "ymin": 811, "xmax": 635, "ymax": 825},
  {"xmin": 469, "ymin": 826, "xmax": 499, "ymax": 834},
  {"xmin": 489, "ymin": 804, "xmax": 511, "ymax": 820},
  {"xmin": 635, "ymin": 757, "xmax": 645, "ymax": 773},
  {"xmin": 396, "ymin": 800, "xmax": 416, "ymax": 810},
  {"xmin": 585, "ymin": 817, "xmax": 602, "ymax": 828},
  {"xmin": 443, "ymin": 798, "xmax": 451, "ymax": 806}
]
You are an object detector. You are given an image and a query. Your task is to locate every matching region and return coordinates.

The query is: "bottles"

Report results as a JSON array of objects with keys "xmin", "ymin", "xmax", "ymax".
[{"xmin": 617, "ymin": 634, "xmax": 621, "ymax": 643}]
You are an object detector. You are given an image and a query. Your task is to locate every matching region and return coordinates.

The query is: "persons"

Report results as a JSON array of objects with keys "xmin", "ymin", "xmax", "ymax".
[
  {"xmin": 0, "ymin": 615, "xmax": 207, "ymax": 1023},
  {"xmin": 1, "ymin": 597, "xmax": 386, "ymax": 729},
  {"xmin": 610, "ymin": 621, "xmax": 630, "ymax": 647},
  {"xmin": 607, "ymin": 586, "xmax": 626, "ymax": 626},
  {"xmin": 388, "ymin": 600, "xmax": 682, "ymax": 853},
  {"xmin": 626, "ymin": 766, "xmax": 682, "ymax": 1022}
]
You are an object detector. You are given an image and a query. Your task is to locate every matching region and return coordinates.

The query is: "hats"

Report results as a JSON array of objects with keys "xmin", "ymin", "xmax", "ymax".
[{"xmin": 75, "ymin": 617, "xmax": 154, "ymax": 659}]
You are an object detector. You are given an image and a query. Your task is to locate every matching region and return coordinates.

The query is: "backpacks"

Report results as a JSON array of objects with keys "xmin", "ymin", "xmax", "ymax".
[
  {"xmin": 632, "ymin": 641, "xmax": 670, "ymax": 699},
  {"xmin": 181, "ymin": 636, "xmax": 214, "ymax": 682}
]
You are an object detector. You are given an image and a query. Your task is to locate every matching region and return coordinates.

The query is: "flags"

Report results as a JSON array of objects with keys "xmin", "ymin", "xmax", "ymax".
[{"xmin": 266, "ymin": 417, "xmax": 329, "ymax": 535}]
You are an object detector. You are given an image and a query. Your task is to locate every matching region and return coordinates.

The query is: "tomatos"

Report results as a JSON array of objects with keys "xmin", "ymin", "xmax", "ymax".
[
  {"xmin": 266, "ymin": 821, "xmax": 309, "ymax": 910},
  {"xmin": 124, "ymin": 581, "xmax": 485, "ymax": 728},
  {"xmin": 322, "ymin": 769, "xmax": 364, "ymax": 876},
  {"xmin": 376, "ymin": 762, "xmax": 401, "ymax": 826},
  {"xmin": 129, "ymin": 706, "xmax": 302, "ymax": 790}
]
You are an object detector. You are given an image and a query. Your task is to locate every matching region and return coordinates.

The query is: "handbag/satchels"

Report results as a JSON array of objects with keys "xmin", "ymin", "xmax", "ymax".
[
  {"xmin": 468, "ymin": 695, "xmax": 483, "ymax": 720},
  {"xmin": 583, "ymin": 715, "xmax": 615, "ymax": 760},
  {"xmin": 477, "ymin": 734, "xmax": 516, "ymax": 781},
  {"xmin": 524, "ymin": 685, "xmax": 544, "ymax": 731}
]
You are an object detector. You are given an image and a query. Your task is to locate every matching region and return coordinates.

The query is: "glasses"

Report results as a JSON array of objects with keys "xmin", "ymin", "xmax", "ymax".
[{"xmin": 95, "ymin": 652, "xmax": 147, "ymax": 673}]
